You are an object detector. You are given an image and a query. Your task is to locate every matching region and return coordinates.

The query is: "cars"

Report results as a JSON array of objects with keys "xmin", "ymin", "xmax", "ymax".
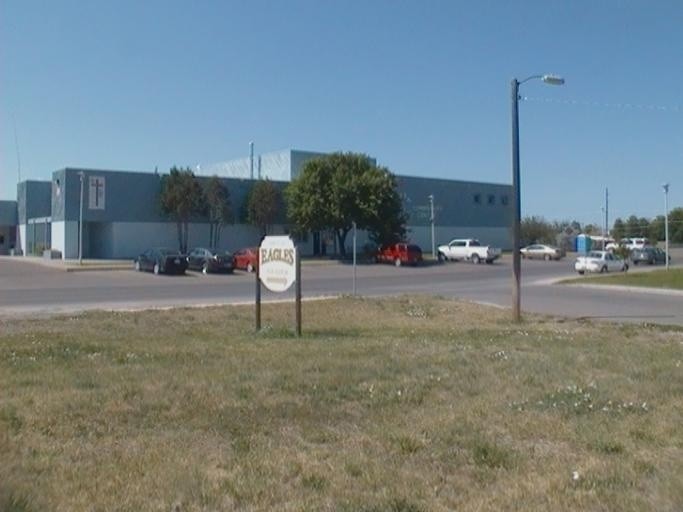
[
  {"xmin": 519, "ymin": 243, "xmax": 567, "ymax": 261},
  {"xmin": 180, "ymin": 245, "xmax": 237, "ymax": 274},
  {"xmin": 229, "ymin": 246, "xmax": 258, "ymax": 272},
  {"xmin": 631, "ymin": 247, "xmax": 672, "ymax": 263},
  {"xmin": 575, "ymin": 249, "xmax": 630, "ymax": 274},
  {"xmin": 133, "ymin": 246, "xmax": 189, "ymax": 275}
]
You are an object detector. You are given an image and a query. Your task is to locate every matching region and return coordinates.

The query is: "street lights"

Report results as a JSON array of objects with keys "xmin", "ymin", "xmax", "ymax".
[
  {"xmin": 76, "ymin": 170, "xmax": 86, "ymax": 267},
  {"xmin": 511, "ymin": 73, "xmax": 566, "ymax": 325},
  {"xmin": 427, "ymin": 194, "xmax": 437, "ymax": 260},
  {"xmin": 598, "ymin": 205, "xmax": 606, "ymax": 252},
  {"xmin": 660, "ymin": 181, "xmax": 672, "ymax": 273}
]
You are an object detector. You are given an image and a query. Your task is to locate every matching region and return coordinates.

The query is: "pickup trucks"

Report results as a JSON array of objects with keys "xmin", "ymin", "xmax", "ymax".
[{"xmin": 438, "ymin": 238, "xmax": 502, "ymax": 263}]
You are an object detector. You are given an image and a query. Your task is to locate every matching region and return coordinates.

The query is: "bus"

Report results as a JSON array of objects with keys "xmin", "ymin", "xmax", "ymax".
[{"xmin": 600, "ymin": 238, "xmax": 651, "ymax": 253}]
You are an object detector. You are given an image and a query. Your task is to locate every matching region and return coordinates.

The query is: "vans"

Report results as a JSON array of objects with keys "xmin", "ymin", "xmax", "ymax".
[{"xmin": 375, "ymin": 243, "xmax": 427, "ymax": 266}]
